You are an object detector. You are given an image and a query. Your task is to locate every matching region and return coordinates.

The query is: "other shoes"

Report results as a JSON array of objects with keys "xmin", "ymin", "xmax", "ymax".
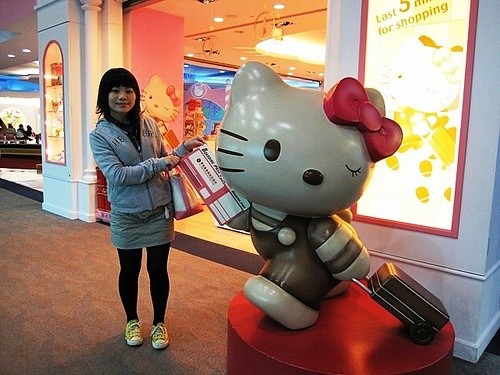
[
  {"xmin": 149, "ymin": 322, "xmax": 170, "ymax": 349},
  {"xmin": 124, "ymin": 320, "xmax": 143, "ymax": 346}
]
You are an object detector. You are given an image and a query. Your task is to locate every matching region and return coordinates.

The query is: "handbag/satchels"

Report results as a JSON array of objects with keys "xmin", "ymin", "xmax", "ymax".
[{"xmin": 168, "ymin": 169, "xmax": 204, "ymax": 220}]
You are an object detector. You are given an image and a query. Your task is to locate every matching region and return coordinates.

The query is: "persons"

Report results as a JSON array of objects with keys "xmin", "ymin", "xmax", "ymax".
[
  {"xmin": 0, "ymin": 118, "xmax": 33, "ymax": 141},
  {"xmin": 89, "ymin": 68, "xmax": 208, "ymax": 349}
]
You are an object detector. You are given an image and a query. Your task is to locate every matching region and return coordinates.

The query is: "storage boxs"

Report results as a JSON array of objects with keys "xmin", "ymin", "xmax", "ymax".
[{"xmin": 178, "ymin": 146, "xmax": 252, "ymax": 227}]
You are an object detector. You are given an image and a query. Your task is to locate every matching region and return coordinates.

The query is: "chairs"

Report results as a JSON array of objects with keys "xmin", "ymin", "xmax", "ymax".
[
  {"xmin": 0, "ymin": 132, "xmax": 4, "ymax": 142},
  {"xmin": 16, "ymin": 131, "xmax": 27, "ymax": 143},
  {"xmin": 3, "ymin": 132, "xmax": 16, "ymax": 143}
]
